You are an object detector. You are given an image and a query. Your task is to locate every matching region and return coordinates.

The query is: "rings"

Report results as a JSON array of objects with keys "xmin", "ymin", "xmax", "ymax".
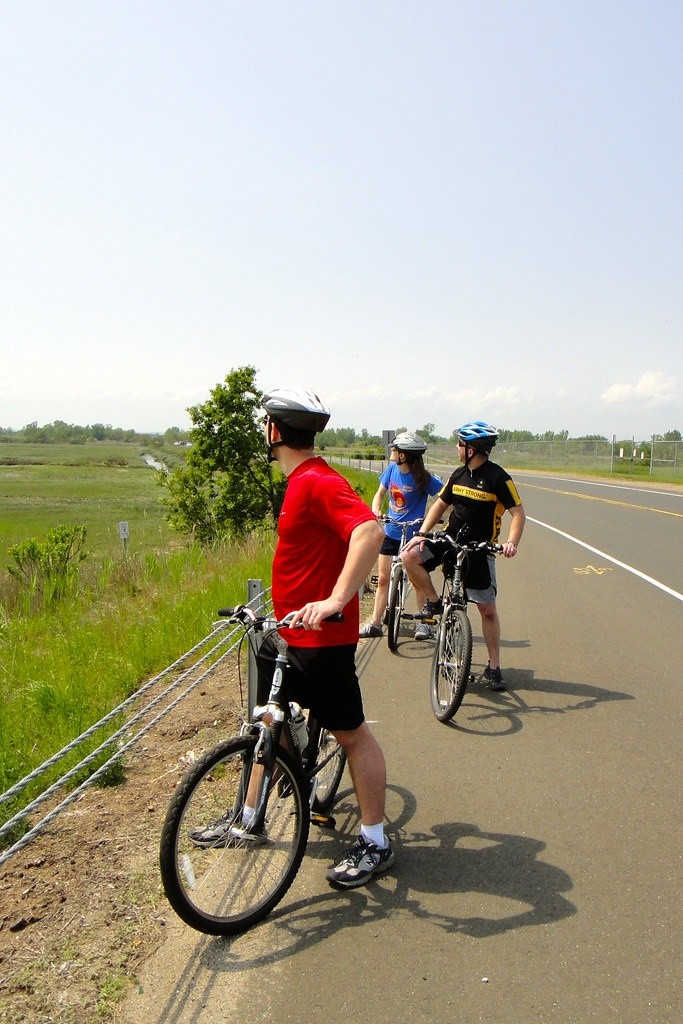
[{"xmin": 309, "ymin": 617, "xmax": 314, "ymax": 621}]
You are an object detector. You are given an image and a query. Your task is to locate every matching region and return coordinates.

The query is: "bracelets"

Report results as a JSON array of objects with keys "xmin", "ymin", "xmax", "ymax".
[{"xmin": 508, "ymin": 541, "xmax": 517, "ymax": 549}]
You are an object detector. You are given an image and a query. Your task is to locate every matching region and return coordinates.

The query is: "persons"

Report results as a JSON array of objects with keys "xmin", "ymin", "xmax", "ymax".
[
  {"xmin": 401, "ymin": 421, "xmax": 525, "ymax": 691},
  {"xmin": 188, "ymin": 384, "xmax": 395, "ymax": 888},
  {"xmin": 359, "ymin": 430, "xmax": 444, "ymax": 639}
]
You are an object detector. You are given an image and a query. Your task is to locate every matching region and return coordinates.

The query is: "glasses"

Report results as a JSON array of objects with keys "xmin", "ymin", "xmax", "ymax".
[
  {"xmin": 458, "ymin": 440, "xmax": 474, "ymax": 450},
  {"xmin": 264, "ymin": 415, "xmax": 270, "ymax": 426}
]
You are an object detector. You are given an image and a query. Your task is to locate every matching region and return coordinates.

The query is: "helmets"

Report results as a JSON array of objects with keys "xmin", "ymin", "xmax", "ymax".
[
  {"xmin": 453, "ymin": 421, "xmax": 500, "ymax": 446},
  {"xmin": 387, "ymin": 432, "xmax": 428, "ymax": 450},
  {"xmin": 261, "ymin": 387, "xmax": 330, "ymax": 433}
]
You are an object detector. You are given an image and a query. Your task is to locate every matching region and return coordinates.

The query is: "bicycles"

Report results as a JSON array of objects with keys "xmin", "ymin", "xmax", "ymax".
[
  {"xmin": 159, "ymin": 605, "xmax": 352, "ymax": 938},
  {"xmin": 371, "ymin": 514, "xmax": 445, "ymax": 651},
  {"xmin": 410, "ymin": 531, "xmax": 506, "ymax": 722}
]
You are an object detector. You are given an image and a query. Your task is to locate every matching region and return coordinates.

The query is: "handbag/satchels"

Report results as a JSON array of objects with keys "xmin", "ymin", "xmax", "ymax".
[{"xmin": 461, "ymin": 549, "xmax": 491, "ymax": 590}]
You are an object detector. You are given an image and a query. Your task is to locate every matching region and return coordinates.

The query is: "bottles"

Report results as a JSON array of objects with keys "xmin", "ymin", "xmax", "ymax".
[{"xmin": 287, "ymin": 702, "xmax": 309, "ymax": 750}]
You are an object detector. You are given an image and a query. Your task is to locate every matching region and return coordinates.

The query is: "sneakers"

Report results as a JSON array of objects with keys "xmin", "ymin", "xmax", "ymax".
[
  {"xmin": 414, "ymin": 598, "xmax": 444, "ymax": 619},
  {"xmin": 413, "ymin": 622, "xmax": 431, "ymax": 640},
  {"xmin": 324, "ymin": 835, "xmax": 394, "ymax": 888},
  {"xmin": 479, "ymin": 660, "xmax": 508, "ymax": 690},
  {"xmin": 359, "ymin": 623, "xmax": 383, "ymax": 637},
  {"xmin": 188, "ymin": 807, "xmax": 269, "ymax": 847}
]
